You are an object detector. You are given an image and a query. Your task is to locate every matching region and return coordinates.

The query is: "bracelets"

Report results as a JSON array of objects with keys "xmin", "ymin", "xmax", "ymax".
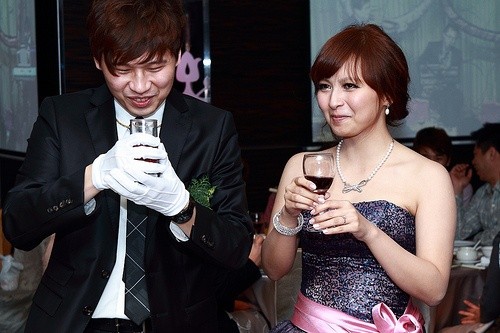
[{"xmin": 273, "ymin": 210, "xmax": 303, "ymax": 236}]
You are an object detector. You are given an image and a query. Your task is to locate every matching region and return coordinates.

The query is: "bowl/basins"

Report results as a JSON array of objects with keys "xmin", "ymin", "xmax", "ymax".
[{"xmin": 481, "ymin": 246, "xmax": 493, "ymax": 257}]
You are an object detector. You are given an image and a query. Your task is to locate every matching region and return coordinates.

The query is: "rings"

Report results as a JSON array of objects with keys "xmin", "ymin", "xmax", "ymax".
[{"xmin": 342, "ymin": 216, "xmax": 346, "ymax": 224}]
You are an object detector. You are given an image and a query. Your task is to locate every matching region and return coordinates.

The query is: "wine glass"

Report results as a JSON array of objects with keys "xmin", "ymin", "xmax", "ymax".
[{"xmin": 303, "ymin": 153, "xmax": 336, "ymax": 232}]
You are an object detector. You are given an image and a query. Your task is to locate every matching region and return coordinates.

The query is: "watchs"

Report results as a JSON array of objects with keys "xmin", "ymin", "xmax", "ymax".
[{"xmin": 171, "ymin": 193, "xmax": 195, "ymax": 224}]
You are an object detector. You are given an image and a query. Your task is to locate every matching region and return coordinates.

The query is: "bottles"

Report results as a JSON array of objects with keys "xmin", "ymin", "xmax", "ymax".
[{"xmin": 456, "ymin": 247, "xmax": 477, "ymax": 260}]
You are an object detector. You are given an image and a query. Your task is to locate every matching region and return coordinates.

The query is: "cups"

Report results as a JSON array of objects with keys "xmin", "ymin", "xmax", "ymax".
[{"xmin": 129, "ymin": 119, "xmax": 160, "ymax": 183}]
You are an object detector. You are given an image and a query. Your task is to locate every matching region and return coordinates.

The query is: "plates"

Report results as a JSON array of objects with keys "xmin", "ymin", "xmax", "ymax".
[{"xmin": 455, "ymin": 261, "xmax": 480, "ymax": 264}]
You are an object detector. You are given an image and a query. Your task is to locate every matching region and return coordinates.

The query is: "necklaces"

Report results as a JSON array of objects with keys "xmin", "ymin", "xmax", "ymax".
[{"xmin": 336, "ymin": 139, "xmax": 394, "ymax": 193}]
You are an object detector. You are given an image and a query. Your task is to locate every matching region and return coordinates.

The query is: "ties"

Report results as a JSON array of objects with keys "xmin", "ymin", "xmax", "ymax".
[{"xmin": 122, "ymin": 114, "xmax": 151, "ymax": 324}]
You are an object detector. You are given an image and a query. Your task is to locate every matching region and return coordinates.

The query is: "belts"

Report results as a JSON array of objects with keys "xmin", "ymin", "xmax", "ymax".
[{"xmin": 86, "ymin": 318, "xmax": 147, "ymax": 333}]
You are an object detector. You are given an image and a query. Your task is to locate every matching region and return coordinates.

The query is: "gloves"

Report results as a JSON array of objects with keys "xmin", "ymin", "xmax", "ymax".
[
  {"xmin": 105, "ymin": 143, "xmax": 189, "ymax": 216},
  {"xmin": 92, "ymin": 133, "xmax": 167, "ymax": 191}
]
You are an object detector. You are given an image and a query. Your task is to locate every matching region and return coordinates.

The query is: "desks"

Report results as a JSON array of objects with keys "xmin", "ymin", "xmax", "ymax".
[{"xmin": 411, "ymin": 263, "xmax": 494, "ymax": 333}]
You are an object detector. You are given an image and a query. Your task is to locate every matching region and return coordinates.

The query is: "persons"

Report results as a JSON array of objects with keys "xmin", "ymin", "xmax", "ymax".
[
  {"xmin": 261, "ymin": 22, "xmax": 457, "ymax": 333},
  {"xmin": 414, "ymin": 122, "xmax": 500, "ymax": 333},
  {"xmin": 3, "ymin": 0, "xmax": 253, "ymax": 333},
  {"xmin": 220, "ymin": 233, "xmax": 266, "ymax": 307}
]
